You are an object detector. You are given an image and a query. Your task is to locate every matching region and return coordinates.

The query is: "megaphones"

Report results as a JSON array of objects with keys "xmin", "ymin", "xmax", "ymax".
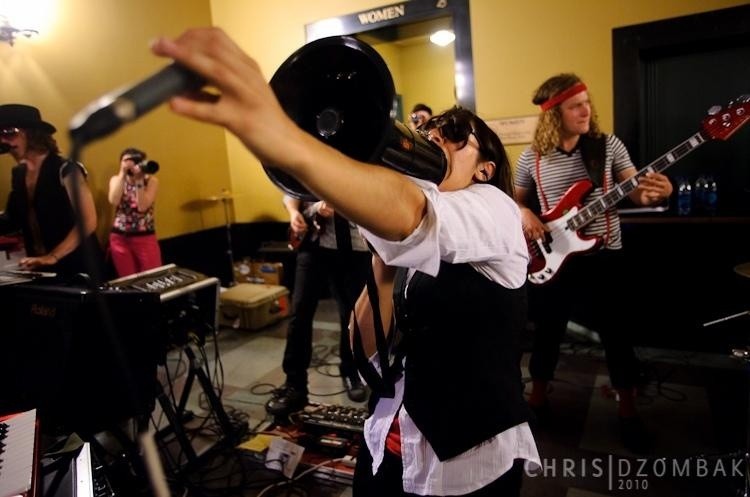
[{"xmin": 256, "ymin": 31, "xmax": 447, "ymax": 203}]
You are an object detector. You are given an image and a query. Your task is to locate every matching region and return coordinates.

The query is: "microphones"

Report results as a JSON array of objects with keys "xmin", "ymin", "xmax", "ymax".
[
  {"xmin": 0, "ymin": 142, "xmax": 12, "ymax": 155},
  {"xmin": 64, "ymin": 56, "xmax": 215, "ymax": 152}
]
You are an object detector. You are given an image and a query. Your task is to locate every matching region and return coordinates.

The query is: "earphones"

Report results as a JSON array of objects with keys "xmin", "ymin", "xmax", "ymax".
[{"xmin": 481, "ymin": 167, "xmax": 489, "ymax": 180}]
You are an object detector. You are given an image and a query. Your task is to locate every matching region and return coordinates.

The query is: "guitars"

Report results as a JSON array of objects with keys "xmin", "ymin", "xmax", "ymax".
[
  {"xmin": 523, "ymin": 91, "xmax": 750, "ymax": 284},
  {"xmin": 288, "ymin": 199, "xmax": 327, "ymax": 250}
]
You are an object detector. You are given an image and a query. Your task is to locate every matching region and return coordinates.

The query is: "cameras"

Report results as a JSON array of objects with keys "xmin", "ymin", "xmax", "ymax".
[{"xmin": 124, "ymin": 151, "xmax": 160, "ymax": 179}]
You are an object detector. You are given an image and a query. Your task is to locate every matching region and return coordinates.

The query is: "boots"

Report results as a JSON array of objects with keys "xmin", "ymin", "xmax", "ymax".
[
  {"xmin": 263, "ymin": 370, "xmax": 309, "ymax": 416},
  {"xmin": 611, "ymin": 384, "xmax": 637, "ymax": 422},
  {"xmin": 526, "ymin": 379, "xmax": 553, "ymax": 417},
  {"xmin": 336, "ymin": 354, "xmax": 367, "ymax": 403}
]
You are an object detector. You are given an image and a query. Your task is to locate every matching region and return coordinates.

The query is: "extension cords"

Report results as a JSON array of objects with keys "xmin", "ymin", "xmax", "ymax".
[{"xmin": 342, "ymin": 455, "xmax": 358, "ymax": 468}]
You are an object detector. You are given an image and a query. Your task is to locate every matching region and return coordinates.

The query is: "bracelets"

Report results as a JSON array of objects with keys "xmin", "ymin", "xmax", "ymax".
[{"xmin": 47, "ymin": 253, "xmax": 57, "ymax": 262}]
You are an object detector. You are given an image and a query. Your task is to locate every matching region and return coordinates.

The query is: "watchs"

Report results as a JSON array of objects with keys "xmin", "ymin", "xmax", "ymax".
[{"xmin": 136, "ymin": 182, "xmax": 145, "ymax": 188}]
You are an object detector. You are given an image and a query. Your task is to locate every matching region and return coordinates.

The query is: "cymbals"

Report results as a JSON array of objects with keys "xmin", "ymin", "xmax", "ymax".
[{"xmin": 199, "ymin": 192, "xmax": 253, "ymax": 202}]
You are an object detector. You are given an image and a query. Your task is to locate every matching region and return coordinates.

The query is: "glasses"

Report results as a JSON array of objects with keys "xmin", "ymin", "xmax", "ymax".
[
  {"xmin": 0, "ymin": 128, "xmax": 20, "ymax": 141},
  {"xmin": 412, "ymin": 121, "xmax": 482, "ymax": 158}
]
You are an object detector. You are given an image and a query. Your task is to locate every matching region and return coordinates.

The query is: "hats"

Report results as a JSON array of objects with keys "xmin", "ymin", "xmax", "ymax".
[{"xmin": 0, "ymin": 104, "xmax": 56, "ymax": 136}]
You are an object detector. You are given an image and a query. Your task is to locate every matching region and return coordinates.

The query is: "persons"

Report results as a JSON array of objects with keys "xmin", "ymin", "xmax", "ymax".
[
  {"xmin": 0, "ymin": 102, "xmax": 119, "ymax": 289},
  {"xmin": 106, "ymin": 146, "xmax": 163, "ymax": 275},
  {"xmin": 150, "ymin": 24, "xmax": 544, "ymax": 495},
  {"xmin": 403, "ymin": 99, "xmax": 434, "ymax": 133},
  {"xmin": 506, "ymin": 68, "xmax": 674, "ymax": 426},
  {"xmin": 265, "ymin": 181, "xmax": 371, "ymax": 413}
]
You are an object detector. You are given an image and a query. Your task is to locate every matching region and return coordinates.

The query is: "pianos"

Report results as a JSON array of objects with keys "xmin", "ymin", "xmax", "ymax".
[{"xmin": 0, "ymin": 407, "xmax": 42, "ymax": 497}]
